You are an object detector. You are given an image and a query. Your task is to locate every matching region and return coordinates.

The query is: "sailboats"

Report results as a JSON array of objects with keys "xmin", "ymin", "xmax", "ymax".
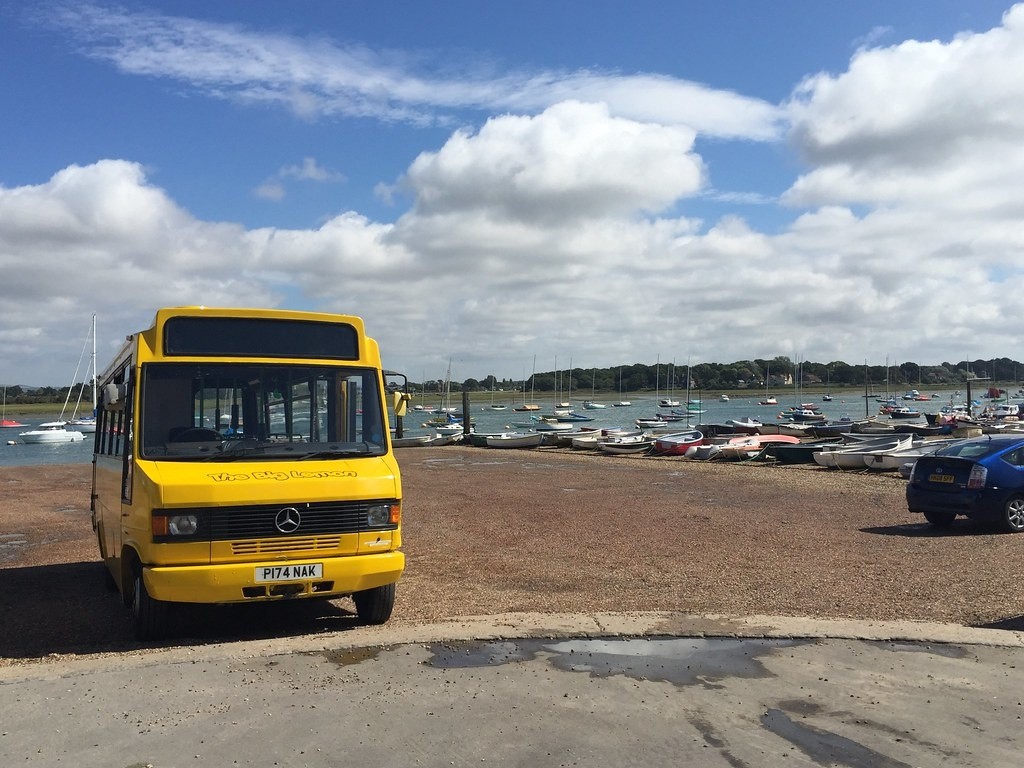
[
  {"xmin": 38, "ymin": 310, "xmax": 101, "ymax": 433},
  {"xmin": 0, "ymin": 384, "xmax": 32, "ymax": 427}
]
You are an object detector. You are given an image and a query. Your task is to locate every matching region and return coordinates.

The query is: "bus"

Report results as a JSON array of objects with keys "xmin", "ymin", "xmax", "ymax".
[{"xmin": 89, "ymin": 306, "xmax": 413, "ymax": 641}]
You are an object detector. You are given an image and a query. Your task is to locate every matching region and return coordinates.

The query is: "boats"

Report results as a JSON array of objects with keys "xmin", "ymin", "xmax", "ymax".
[
  {"xmin": 194, "ymin": 415, "xmax": 207, "ymax": 420},
  {"xmin": 387, "ymin": 349, "xmax": 1024, "ymax": 476},
  {"xmin": 18, "ymin": 427, "xmax": 88, "ymax": 444}
]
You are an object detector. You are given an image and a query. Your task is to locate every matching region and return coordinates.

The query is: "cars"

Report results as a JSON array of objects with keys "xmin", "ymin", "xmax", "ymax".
[{"xmin": 906, "ymin": 434, "xmax": 1024, "ymax": 532}]
